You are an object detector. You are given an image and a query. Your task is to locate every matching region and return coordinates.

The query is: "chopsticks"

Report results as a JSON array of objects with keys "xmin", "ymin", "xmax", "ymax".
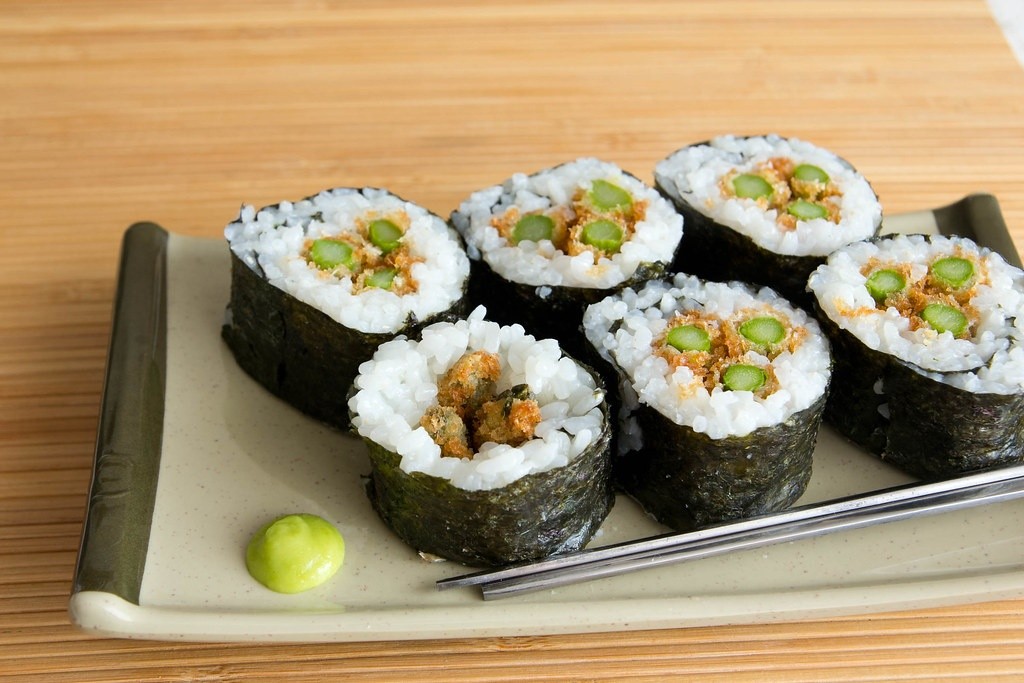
[{"xmin": 432, "ymin": 460, "xmax": 1024, "ymax": 603}]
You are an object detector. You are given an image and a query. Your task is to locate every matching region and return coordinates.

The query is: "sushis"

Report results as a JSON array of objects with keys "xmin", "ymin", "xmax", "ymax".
[{"xmin": 220, "ymin": 133, "xmax": 1024, "ymax": 571}]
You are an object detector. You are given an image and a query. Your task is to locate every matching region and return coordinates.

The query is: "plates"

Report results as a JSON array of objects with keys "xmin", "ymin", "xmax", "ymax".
[{"xmin": 67, "ymin": 192, "xmax": 1024, "ymax": 641}]
